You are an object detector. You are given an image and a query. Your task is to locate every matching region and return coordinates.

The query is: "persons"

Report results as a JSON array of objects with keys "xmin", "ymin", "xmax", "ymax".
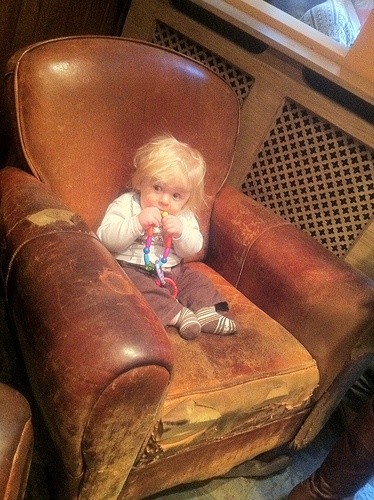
[{"xmin": 97, "ymin": 132, "xmax": 237, "ymax": 340}]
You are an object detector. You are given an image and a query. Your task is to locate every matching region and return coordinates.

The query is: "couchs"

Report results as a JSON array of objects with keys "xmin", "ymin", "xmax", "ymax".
[
  {"xmin": 0, "ymin": 383, "xmax": 36, "ymax": 500},
  {"xmin": 0, "ymin": 34, "xmax": 374, "ymax": 500}
]
